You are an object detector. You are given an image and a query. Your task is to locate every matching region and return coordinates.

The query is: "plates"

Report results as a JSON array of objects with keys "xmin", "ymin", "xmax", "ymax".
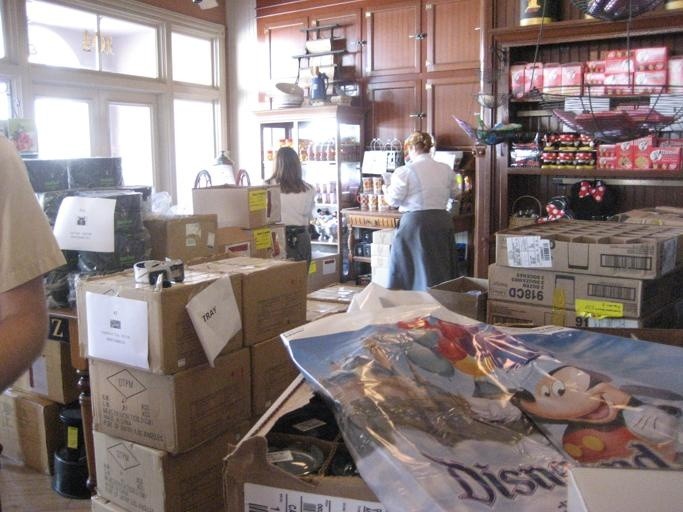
[{"xmin": 279, "ymin": 99, "xmax": 303, "ymax": 110}]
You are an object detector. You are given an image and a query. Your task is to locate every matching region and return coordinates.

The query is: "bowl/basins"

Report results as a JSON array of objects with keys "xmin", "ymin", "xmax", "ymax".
[{"xmin": 274, "ymin": 82, "xmax": 304, "ymax": 103}]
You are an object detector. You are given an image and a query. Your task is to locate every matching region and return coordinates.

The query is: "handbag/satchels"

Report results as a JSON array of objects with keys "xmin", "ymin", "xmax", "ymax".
[{"xmin": 361, "ymin": 150, "xmax": 404, "ymax": 174}]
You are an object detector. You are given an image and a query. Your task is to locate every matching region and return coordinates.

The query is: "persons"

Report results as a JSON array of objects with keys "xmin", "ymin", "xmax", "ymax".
[
  {"xmin": 381, "ymin": 131, "xmax": 460, "ymax": 290},
  {"xmin": 265, "ymin": 147, "xmax": 316, "ymax": 271},
  {"xmin": 0, "ymin": 131, "xmax": 68, "ymax": 396}
]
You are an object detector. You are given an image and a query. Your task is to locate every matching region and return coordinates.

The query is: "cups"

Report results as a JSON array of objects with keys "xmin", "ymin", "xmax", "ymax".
[
  {"xmin": 371, "ymin": 177, "xmax": 384, "ymax": 194},
  {"xmin": 377, "ymin": 195, "xmax": 389, "ymax": 211},
  {"xmin": 361, "ymin": 177, "xmax": 372, "ymax": 194},
  {"xmin": 356, "ymin": 194, "xmax": 367, "ymax": 210},
  {"xmin": 367, "ymin": 194, "xmax": 377, "ymax": 210}
]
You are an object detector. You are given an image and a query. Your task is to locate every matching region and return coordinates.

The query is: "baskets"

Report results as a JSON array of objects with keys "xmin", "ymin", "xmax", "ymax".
[{"xmin": 510, "ymin": 195, "xmax": 542, "ymax": 228}]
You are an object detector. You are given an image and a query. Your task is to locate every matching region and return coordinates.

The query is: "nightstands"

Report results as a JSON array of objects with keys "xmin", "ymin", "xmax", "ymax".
[{"xmin": 427, "ymin": 267, "xmax": 682, "ymax": 329}]
